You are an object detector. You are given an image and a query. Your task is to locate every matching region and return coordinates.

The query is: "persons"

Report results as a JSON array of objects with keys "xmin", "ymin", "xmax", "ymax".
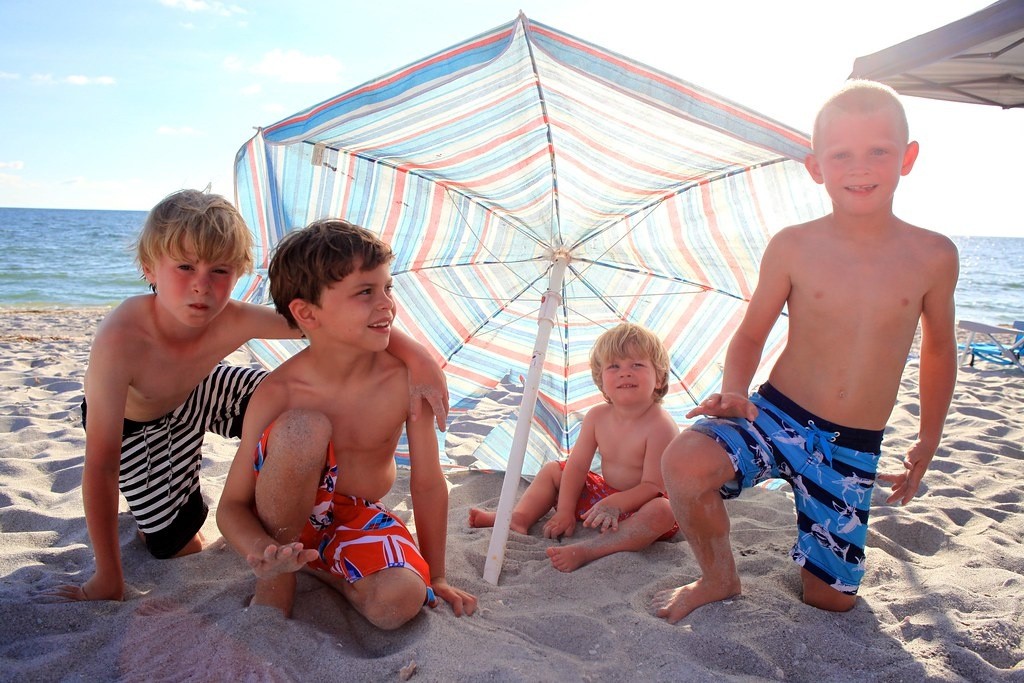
[
  {"xmin": 215, "ymin": 219, "xmax": 479, "ymax": 628},
  {"xmin": 468, "ymin": 324, "xmax": 682, "ymax": 575},
  {"xmin": 648, "ymin": 87, "xmax": 960, "ymax": 626},
  {"xmin": 47, "ymin": 189, "xmax": 449, "ymax": 605}
]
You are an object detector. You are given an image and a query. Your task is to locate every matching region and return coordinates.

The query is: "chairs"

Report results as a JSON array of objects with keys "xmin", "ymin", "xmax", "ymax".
[{"xmin": 956, "ymin": 321, "xmax": 1024, "ymax": 372}]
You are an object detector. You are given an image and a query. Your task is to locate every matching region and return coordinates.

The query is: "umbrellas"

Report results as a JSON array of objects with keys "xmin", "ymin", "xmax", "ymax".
[
  {"xmin": 230, "ymin": 11, "xmax": 831, "ymax": 586},
  {"xmin": 849, "ymin": 0, "xmax": 1024, "ymax": 111}
]
